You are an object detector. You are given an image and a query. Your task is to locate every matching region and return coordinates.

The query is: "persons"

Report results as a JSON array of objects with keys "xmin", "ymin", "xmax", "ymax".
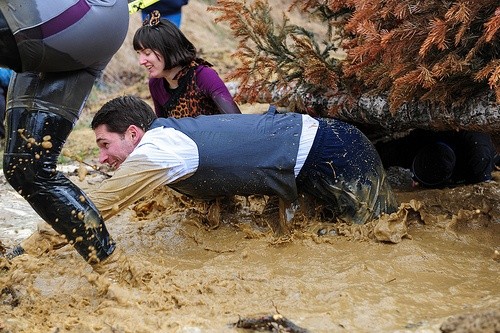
[
  {"xmin": 133, "ymin": 20, "xmax": 240, "ymax": 117},
  {"xmin": 142, "ymin": 0, "xmax": 188, "ymax": 31},
  {"xmin": 0, "ymin": 0, "xmax": 130, "ymax": 283},
  {"xmin": 3, "ymin": 96, "xmax": 401, "ymax": 266}
]
